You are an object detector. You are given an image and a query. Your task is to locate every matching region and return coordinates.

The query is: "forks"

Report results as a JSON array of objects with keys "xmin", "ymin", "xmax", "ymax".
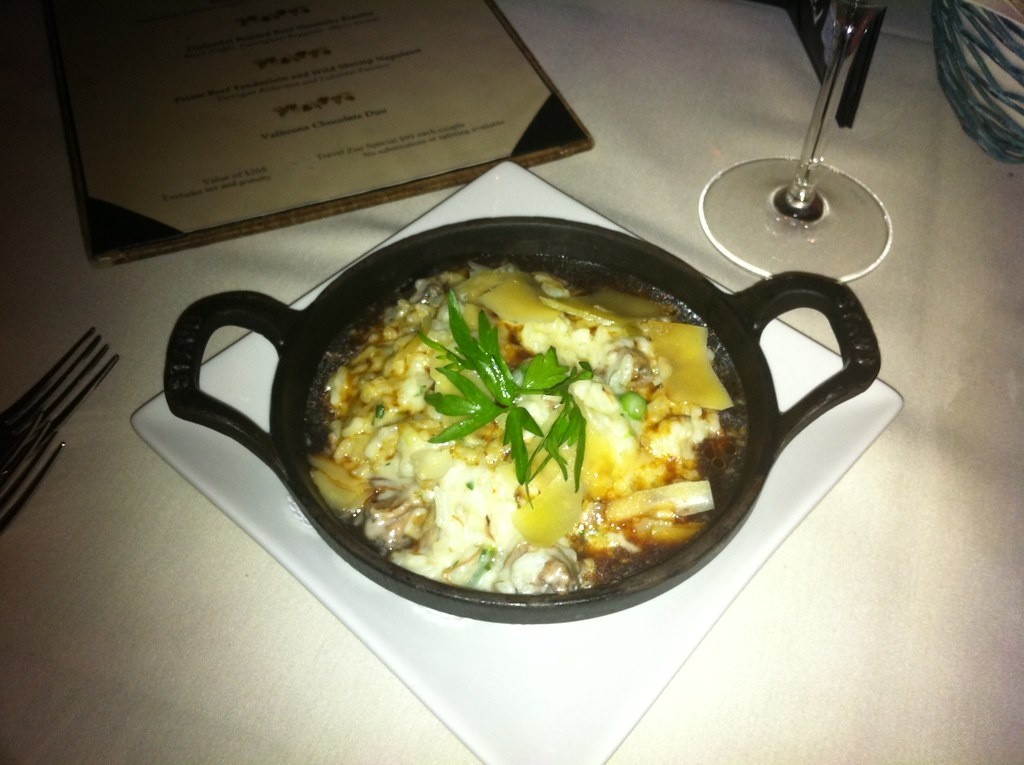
[{"xmin": 0, "ymin": 327, "xmax": 120, "ymax": 537}]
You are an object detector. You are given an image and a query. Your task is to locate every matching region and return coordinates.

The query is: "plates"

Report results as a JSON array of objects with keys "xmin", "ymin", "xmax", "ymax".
[{"xmin": 130, "ymin": 160, "xmax": 904, "ymax": 765}]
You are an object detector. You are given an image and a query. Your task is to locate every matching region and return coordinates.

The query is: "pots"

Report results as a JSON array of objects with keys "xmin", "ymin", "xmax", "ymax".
[{"xmin": 164, "ymin": 216, "xmax": 882, "ymax": 625}]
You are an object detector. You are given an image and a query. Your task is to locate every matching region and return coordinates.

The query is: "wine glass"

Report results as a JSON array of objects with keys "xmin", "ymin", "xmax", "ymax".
[{"xmin": 703, "ymin": 0, "xmax": 893, "ymax": 277}]
